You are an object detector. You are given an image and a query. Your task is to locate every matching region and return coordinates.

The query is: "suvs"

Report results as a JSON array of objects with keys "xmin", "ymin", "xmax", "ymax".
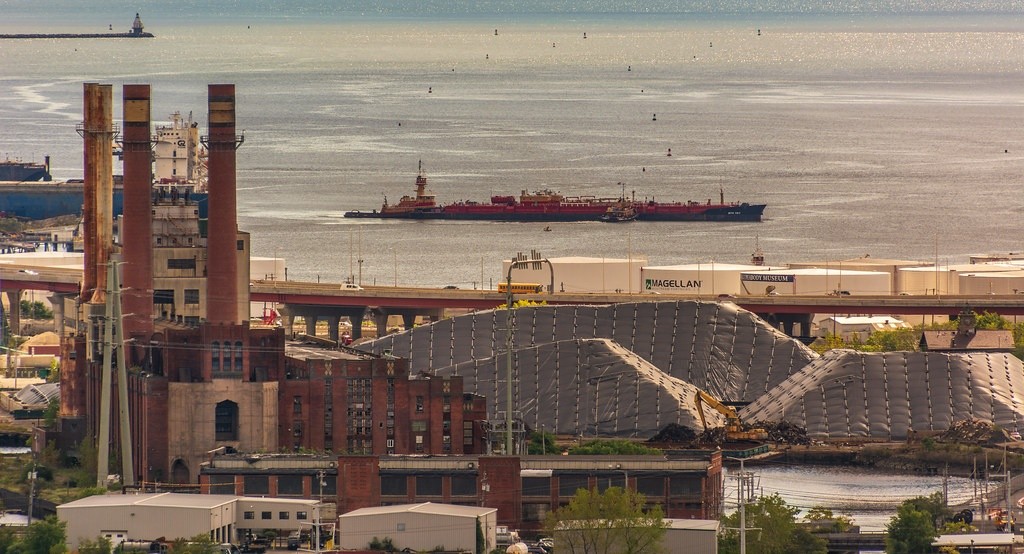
[{"xmin": 247, "ymin": 534, "xmax": 257, "ymax": 541}]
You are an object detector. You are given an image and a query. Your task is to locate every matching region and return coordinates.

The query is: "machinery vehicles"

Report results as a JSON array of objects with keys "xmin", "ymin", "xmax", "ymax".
[{"xmin": 693, "ymin": 392, "xmax": 767, "ymax": 443}]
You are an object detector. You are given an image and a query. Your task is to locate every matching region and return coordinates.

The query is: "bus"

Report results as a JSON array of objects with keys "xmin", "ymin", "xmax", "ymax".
[{"xmin": 498, "ymin": 283, "xmax": 544, "ymax": 294}]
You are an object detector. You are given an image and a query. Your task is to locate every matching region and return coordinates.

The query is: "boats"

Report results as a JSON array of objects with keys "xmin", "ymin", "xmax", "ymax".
[
  {"xmin": 604, "ymin": 196, "xmax": 639, "ymax": 222},
  {"xmin": 750, "ymin": 233, "xmax": 764, "ymax": 265},
  {"xmin": 346, "ymin": 156, "xmax": 768, "ymax": 223}
]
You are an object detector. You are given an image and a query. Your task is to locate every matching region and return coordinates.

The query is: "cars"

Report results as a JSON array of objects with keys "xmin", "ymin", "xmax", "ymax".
[{"xmin": 899, "ymin": 292, "xmax": 913, "ymax": 295}]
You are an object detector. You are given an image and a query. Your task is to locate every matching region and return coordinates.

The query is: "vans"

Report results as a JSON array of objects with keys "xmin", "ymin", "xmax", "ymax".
[
  {"xmin": 340, "ymin": 283, "xmax": 363, "ymax": 291},
  {"xmin": 836, "ymin": 290, "xmax": 850, "ymax": 296}
]
